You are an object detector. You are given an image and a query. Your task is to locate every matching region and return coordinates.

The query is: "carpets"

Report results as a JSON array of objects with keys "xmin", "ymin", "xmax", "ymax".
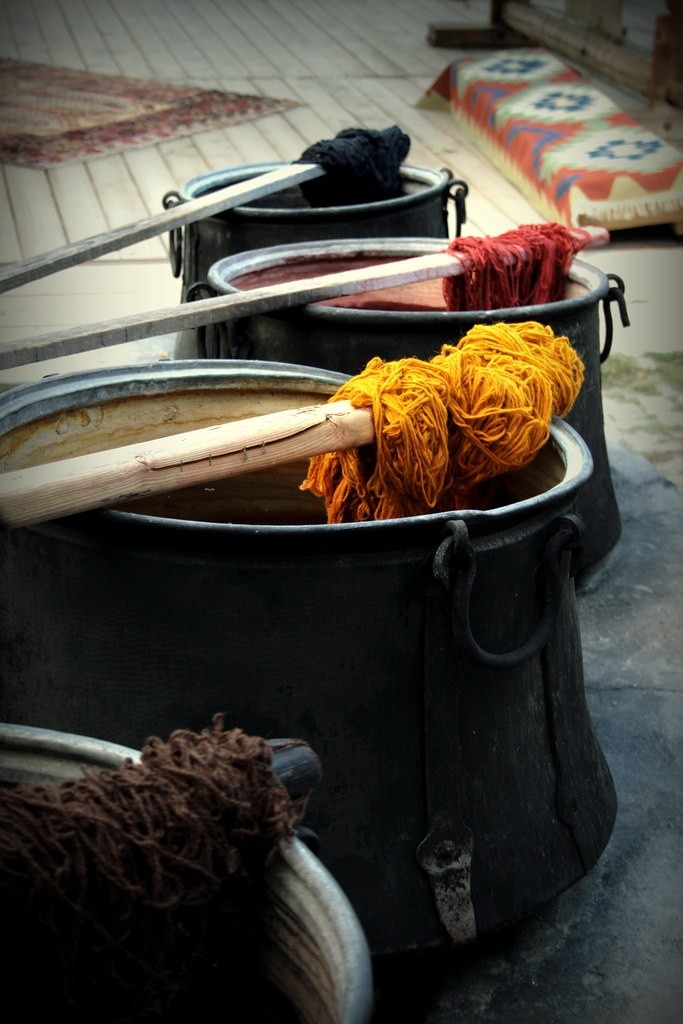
[{"xmin": 0, "ymin": 58, "xmax": 308, "ymax": 171}]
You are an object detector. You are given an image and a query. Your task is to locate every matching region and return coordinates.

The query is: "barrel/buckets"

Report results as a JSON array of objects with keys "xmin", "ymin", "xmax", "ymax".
[
  {"xmin": 162, "ymin": 159, "xmax": 465, "ymax": 359},
  {"xmin": 186, "ymin": 237, "xmax": 623, "ymax": 571},
  {"xmin": 0, "ymin": 360, "xmax": 606, "ymax": 957},
  {"xmin": 0, "ymin": 724, "xmax": 369, "ymax": 1024}
]
